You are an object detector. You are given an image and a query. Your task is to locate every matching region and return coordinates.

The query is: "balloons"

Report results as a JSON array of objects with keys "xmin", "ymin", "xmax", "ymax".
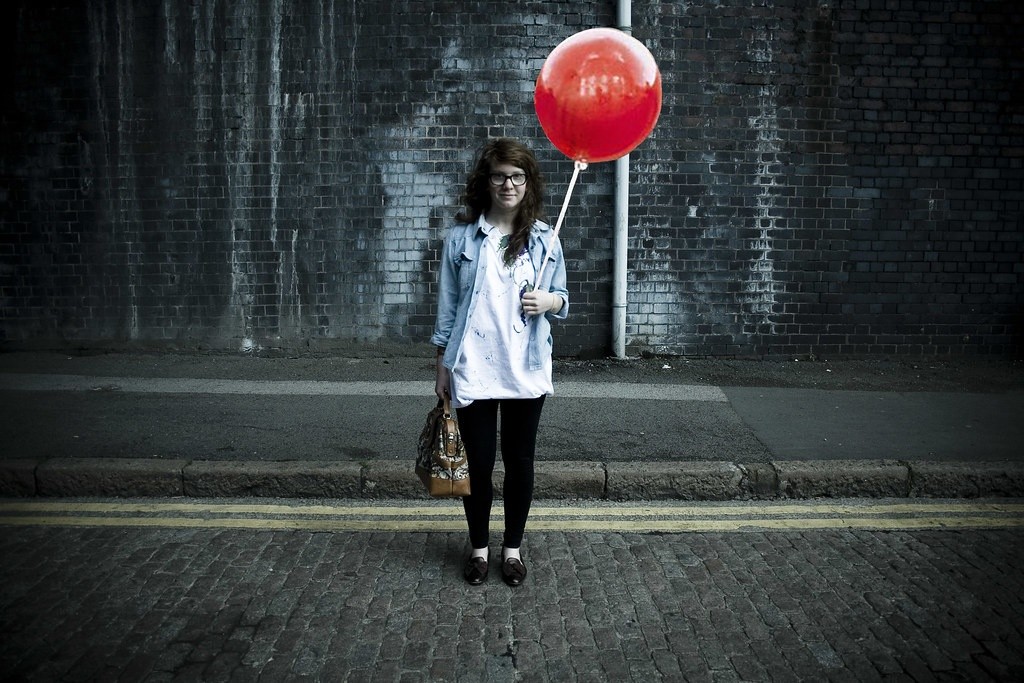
[{"xmin": 534, "ymin": 28, "xmax": 663, "ymax": 162}]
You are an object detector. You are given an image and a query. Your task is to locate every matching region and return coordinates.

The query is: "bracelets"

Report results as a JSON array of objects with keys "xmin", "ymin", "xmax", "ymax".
[{"xmin": 549, "ymin": 293, "xmax": 555, "ymax": 311}]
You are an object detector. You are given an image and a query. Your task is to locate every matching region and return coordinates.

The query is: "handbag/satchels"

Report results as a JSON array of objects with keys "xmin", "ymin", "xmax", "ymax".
[{"xmin": 415, "ymin": 391, "xmax": 471, "ymax": 497}]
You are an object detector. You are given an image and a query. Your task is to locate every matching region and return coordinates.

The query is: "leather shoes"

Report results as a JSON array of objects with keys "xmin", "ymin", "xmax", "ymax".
[
  {"xmin": 464, "ymin": 546, "xmax": 491, "ymax": 586},
  {"xmin": 501, "ymin": 547, "xmax": 526, "ymax": 586}
]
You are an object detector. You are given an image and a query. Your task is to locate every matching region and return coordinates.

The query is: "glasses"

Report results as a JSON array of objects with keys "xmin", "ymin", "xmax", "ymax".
[{"xmin": 488, "ymin": 173, "xmax": 529, "ymax": 186}]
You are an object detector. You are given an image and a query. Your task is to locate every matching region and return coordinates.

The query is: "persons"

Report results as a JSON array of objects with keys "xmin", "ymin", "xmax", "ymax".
[{"xmin": 430, "ymin": 140, "xmax": 570, "ymax": 587}]
